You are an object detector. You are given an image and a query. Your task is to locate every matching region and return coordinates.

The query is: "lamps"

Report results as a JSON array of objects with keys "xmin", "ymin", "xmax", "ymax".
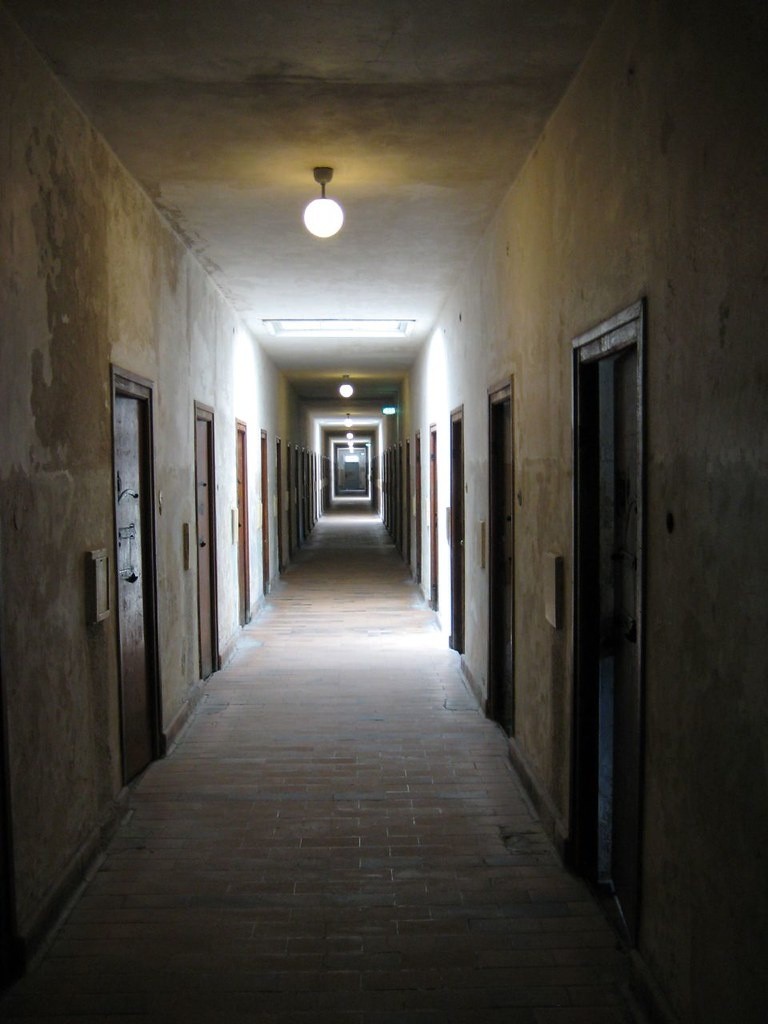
[
  {"xmin": 338, "ymin": 374, "xmax": 355, "ymax": 396},
  {"xmin": 344, "ymin": 412, "xmax": 353, "ymax": 428},
  {"xmin": 303, "ymin": 164, "xmax": 344, "ymax": 239}
]
